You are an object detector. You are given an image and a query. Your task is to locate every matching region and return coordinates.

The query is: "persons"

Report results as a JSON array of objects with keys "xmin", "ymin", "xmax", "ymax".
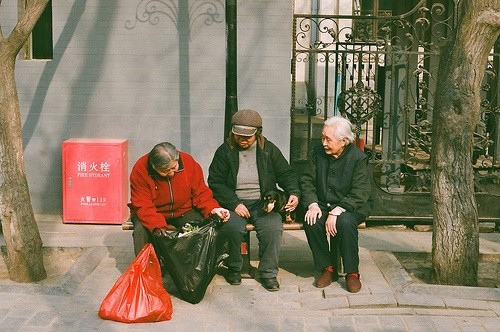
[
  {"xmin": 207, "ymin": 110, "xmax": 302, "ymax": 292},
  {"xmin": 295, "ymin": 116, "xmax": 376, "ymax": 293},
  {"xmin": 127, "ymin": 142, "xmax": 230, "ymax": 276}
]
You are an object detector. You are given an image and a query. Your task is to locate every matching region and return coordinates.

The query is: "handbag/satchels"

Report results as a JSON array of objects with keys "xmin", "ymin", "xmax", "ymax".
[
  {"xmin": 152, "ymin": 213, "xmax": 221, "ymax": 304},
  {"xmin": 99, "ymin": 244, "xmax": 173, "ymax": 322}
]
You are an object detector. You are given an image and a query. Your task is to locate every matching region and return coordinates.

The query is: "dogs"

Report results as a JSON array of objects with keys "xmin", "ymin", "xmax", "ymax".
[{"xmin": 261, "ymin": 187, "xmax": 303, "ymax": 223}]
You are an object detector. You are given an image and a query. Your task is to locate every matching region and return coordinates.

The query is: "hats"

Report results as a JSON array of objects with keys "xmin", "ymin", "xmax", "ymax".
[{"xmin": 226, "ymin": 108, "xmax": 264, "ymax": 151}]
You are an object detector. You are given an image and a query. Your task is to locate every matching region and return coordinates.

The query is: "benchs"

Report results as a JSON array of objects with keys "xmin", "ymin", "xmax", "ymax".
[{"xmin": 121, "ymin": 198, "xmax": 366, "ymax": 274}]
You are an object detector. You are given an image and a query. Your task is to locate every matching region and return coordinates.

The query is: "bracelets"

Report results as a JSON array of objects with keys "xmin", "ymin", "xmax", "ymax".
[{"xmin": 328, "ymin": 212, "xmax": 340, "ymax": 216}]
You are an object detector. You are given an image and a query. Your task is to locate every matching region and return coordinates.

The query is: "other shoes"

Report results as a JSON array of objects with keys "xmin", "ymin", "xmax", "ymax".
[
  {"xmin": 261, "ymin": 277, "xmax": 280, "ymax": 291},
  {"xmin": 316, "ymin": 266, "xmax": 339, "ymax": 288},
  {"xmin": 344, "ymin": 273, "xmax": 362, "ymax": 293},
  {"xmin": 226, "ymin": 270, "xmax": 242, "ymax": 285}
]
